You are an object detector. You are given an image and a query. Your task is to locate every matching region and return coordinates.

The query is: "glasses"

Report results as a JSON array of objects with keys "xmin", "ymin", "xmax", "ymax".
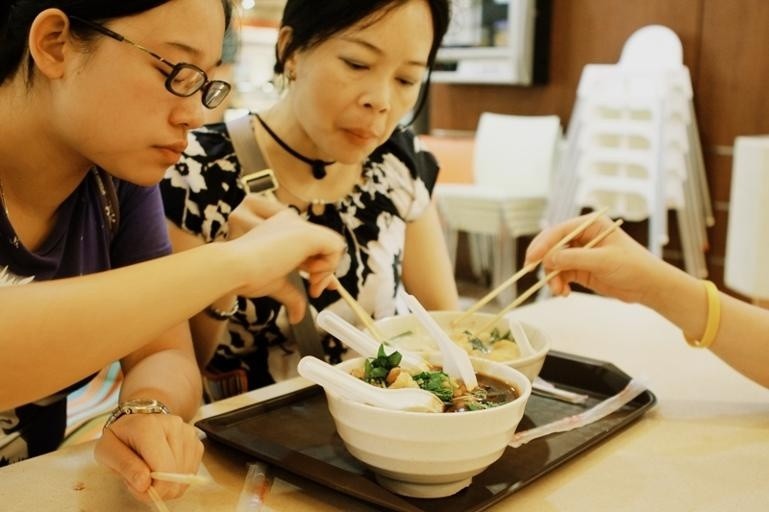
[{"xmin": 96, "ymin": 21, "xmax": 231, "ymax": 109}]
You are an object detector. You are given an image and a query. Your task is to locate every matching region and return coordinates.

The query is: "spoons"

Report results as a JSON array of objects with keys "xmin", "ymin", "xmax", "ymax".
[
  {"xmin": 298, "ymin": 355, "xmax": 445, "ymax": 416},
  {"xmin": 317, "ymin": 310, "xmax": 423, "ymax": 374},
  {"xmin": 404, "ymin": 294, "xmax": 478, "ymax": 390}
]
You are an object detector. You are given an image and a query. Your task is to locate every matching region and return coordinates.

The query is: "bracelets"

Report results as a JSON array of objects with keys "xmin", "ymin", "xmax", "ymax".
[
  {"xmin": 684, "ymin": 278, "xmax": 720, "ymax": 349},
  {"xmin": 203, "ymin": 301, "xmax": 239, "ymax": 321}
]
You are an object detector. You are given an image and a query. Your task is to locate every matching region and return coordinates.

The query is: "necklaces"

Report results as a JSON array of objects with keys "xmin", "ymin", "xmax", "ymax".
[{"xmin": 249, "ymin": 112, "xmax": 337, "ymax": 180}]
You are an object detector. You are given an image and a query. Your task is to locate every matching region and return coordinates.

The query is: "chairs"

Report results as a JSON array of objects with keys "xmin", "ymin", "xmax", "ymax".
[{"xmin": 432, "ymin": 110, "xmax": 565, "ymax": 308}]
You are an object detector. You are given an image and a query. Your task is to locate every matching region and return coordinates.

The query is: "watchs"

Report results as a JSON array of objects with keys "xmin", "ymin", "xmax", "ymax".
[{"xmin": 102, "ymin": 398, "xmax": 170, "ymax": 430}]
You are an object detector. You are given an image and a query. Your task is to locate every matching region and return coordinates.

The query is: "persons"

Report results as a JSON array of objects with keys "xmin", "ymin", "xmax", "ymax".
[
  {"xmin": 522, "ymin": 213, "xmax": 768, "ymax": 388},
  {"xmin": 0, "ymin": 0, "xmax": 349, "ymax": 505},
  {"xmin": 157, "ymin": 0, "xmax": 463, "ymax": 408}
]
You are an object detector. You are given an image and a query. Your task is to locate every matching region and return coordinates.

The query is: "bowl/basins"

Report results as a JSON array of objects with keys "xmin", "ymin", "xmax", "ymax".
[
  {"xmin": 362, "ymin": 311, "xmax": 549, "ymax": 386},
  {"xmin": 325, "ymin": 356, "xmax": 532, "ymax": 498}
]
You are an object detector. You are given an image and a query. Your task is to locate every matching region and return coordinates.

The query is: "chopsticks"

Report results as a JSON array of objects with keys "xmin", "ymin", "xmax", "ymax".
[
  {"xmin": 451, "ymin": 208, "xmax": 622, "ymax": 337},
  {"xmin": 144, "ymin": 470, "xmax": 209, "ymax": 512},
  {"xmin": 333, "ymin": 273, "xmax": 388, "ymax": 347}
]
